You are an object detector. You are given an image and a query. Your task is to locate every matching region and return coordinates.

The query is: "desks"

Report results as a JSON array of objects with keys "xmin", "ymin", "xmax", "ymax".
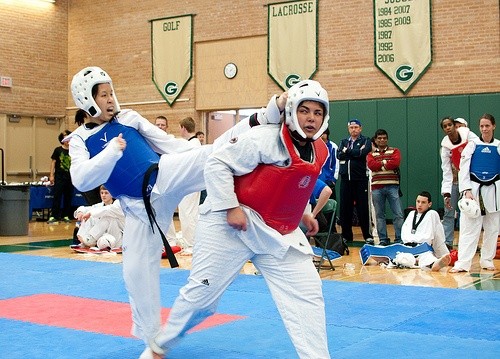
[{"xmin": 28, "ymin": 185, "xmax": 54, "ymax": 220}]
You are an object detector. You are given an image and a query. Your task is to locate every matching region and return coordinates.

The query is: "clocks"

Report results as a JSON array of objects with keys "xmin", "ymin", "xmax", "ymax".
[{"xmin": 224, "ymin": 63, "xmax": 238, "ymax": 79}]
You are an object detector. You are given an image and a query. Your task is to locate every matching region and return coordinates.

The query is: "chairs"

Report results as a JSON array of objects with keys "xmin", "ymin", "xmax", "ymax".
[{"xmin": 307, "ymin": 199, "xmax": 338, "ymax": 273}]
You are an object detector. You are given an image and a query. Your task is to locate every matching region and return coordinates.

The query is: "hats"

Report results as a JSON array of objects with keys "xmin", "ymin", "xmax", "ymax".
[
  {"xmin": 348, "ymin": 119, "xmax": 361, "ymax": 127},
  {"xmin": 454, "ymin": 118, "xmax": 468, "ymax": 127}
]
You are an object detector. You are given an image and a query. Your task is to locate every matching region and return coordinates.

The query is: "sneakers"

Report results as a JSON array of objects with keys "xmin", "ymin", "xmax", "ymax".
[
  {"xmin": 344, "ymin": 238, "xmax": 353, "ymax": 243},
  {"xmin": 379, "ymin": 239, "xmax": 390, "ymax": 246},
  {"xmin": 394, "ymin": 239, "xmax": 402, "ymax": 244},
  {"xmin": 47, "ymin": 217, "xmax": 57, "ymax": 223},
  {"xmin": 365, "ymin": 238, "xmax": 375, "ymax": 244},
  {"xmin": 64, "ymin": 217, "xmax": 72, "ymax": 223}
]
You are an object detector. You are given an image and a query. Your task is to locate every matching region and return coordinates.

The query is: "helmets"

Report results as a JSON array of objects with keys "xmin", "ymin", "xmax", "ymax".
[
  {"xmin": 285, "ymin": 80, "xmax": 330, "ymax": 141},
  {"xmin": 458, "ymin": 198, "xmax": 481, "ymax": 219},
  {"xmin": 70, "ymin": 67, "xmax": 120, "ymax": 117},
  {"xmin": 97, "ymin": 234, "xmax": 117, "ymax": 249}
]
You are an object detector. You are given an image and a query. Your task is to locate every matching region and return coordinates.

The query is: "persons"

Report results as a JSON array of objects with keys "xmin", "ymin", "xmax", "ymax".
[
  {"xmin": 337, "ymin": 119, "xmax": 376, "ymax": 244},
  {"xmin": 46, "ymin": 130, "xmax": 74, "ymax": 223},
  {"xmin": 299, "ymin": 128, "xmax": 340, "ymax": 243},
  {"xmin": 62, "ymin": 66, "xmax": 289, "ymax": 339},
  {"xmin": 440, "ymin": 116, "xmax": 479, "ymax": 252},
  {"xmin": 366, "ymin": 129, "xmax": 404, "ymax": 246},
  {"xmin": 70, "ymin": 108, "xmax": 207, "ymax": 253},
  {"xmin": 393, "ymin": 191, "xmax": 451, "ymax": 272},
  {"xmin": 139, "ymin": 80, "xmax": 330, "ymax": 359},
  {"xmin": 449, "ymin": 114, "xmax": 500, "ymax": 272}
]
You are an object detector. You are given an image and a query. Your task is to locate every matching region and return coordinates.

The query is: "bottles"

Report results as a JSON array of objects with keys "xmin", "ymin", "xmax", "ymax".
[{"xmin": 342, "ymin": 264, "xmax": 355, "ymax": 269}]
